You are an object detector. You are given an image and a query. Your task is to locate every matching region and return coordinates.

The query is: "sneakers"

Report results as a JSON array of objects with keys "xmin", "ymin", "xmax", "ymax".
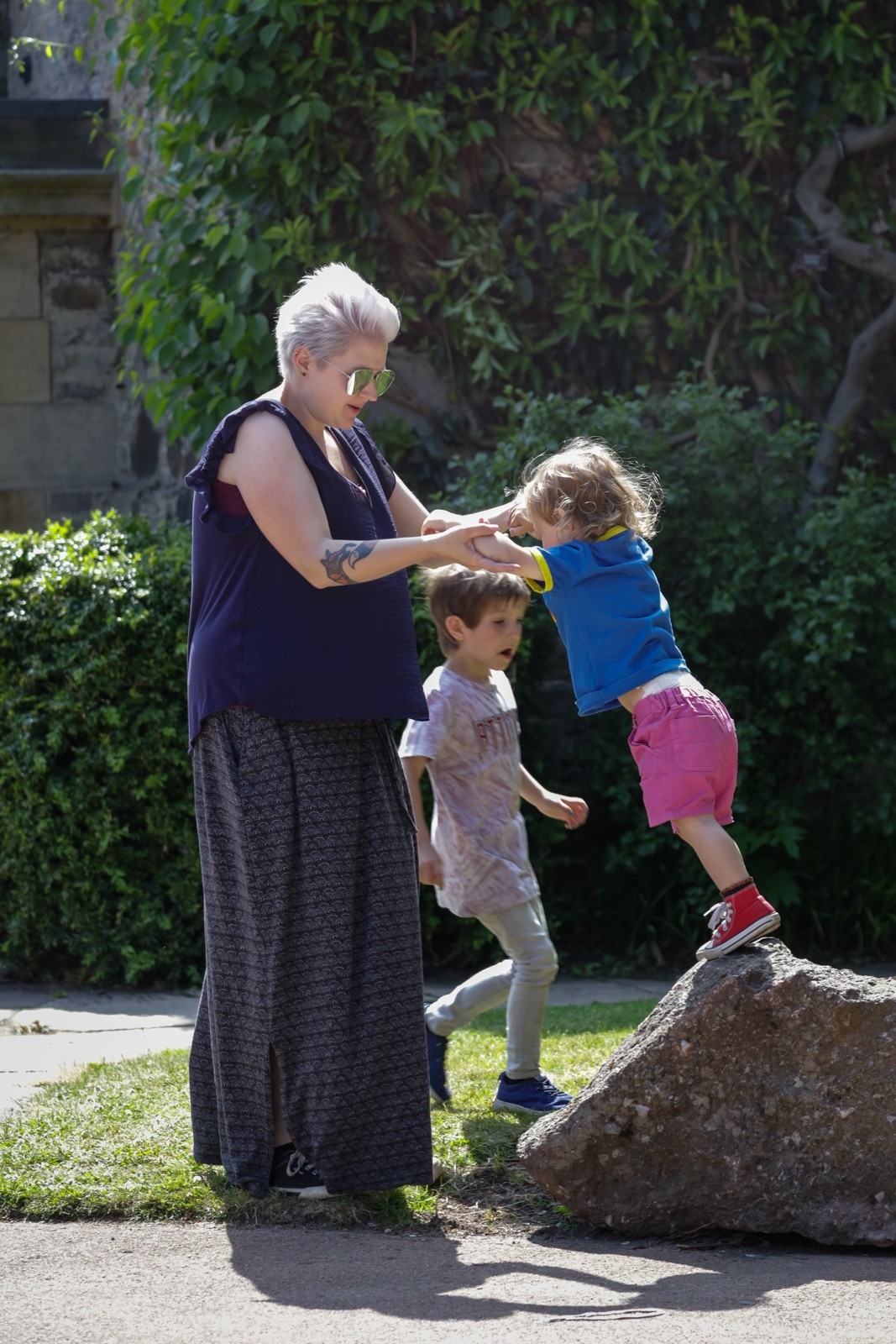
[
  {"xmin": 492, "ymin": 1071, "xmax": 574, "ymax": 1116},
  {"xmin": 695, "ymin": 883, "xmax": 782, "ymax": 962},
  {"xmin": 423, "ymin": 1002, "xmax": 451, "ymax": 1104},
  {"xmin": 268, "ymin": 1148, "xmax": 346, "ymax": 1198}
]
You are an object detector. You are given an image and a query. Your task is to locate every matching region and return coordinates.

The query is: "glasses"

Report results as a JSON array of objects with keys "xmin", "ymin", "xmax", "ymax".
[{"xmin": 308, "ymin": 348, "xmax": 396, "ymax": 397}]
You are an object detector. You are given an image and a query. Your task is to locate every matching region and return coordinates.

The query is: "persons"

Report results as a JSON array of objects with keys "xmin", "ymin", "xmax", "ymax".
[
  {"xmin": 396, "ymin": 562, "xmax": 588, "ymax": 1116},
  {"xmin": 421, "ymin": 436, "xmax": 780, "ymax": 960},
  {"xmin": 185, "ymin": 262, "xmax": 528, "ymax": 1199}
]
